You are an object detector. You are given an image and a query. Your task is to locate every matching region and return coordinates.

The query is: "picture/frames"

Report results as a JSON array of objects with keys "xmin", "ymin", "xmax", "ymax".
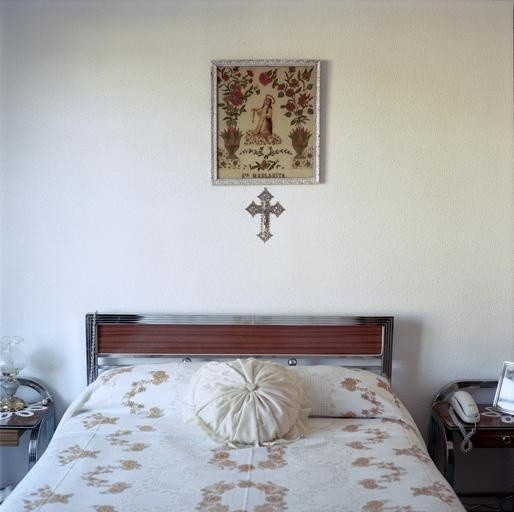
[
  {"xmin": 493, "ymin": 361, "xmax": 514, "ymax": 416},
  {"xmin": 210, "ymin": 59, "xmax": 321, "ymax": 185}
]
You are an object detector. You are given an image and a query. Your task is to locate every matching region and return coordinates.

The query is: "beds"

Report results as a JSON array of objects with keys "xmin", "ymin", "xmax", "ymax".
[{"xmin": 0, "ymin": 315, "xmax": 468, "ymax": 511}]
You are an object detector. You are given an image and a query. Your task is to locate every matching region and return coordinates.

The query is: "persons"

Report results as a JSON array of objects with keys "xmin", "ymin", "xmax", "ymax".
[{"xmin": 250, "ymin": 93, "xmax": 276, "ymax": 136}]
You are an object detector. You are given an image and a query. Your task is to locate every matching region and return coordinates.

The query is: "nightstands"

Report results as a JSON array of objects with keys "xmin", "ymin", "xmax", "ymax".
[
  {"xmin": 0, "ymin": 378, "xmax": 55, "ymax": 471},
  {"xmin": 428, "ymin": 378, "xmax": 514, "ymax": 488}
]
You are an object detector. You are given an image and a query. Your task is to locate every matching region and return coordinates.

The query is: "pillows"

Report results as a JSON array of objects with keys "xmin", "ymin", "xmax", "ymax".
[{"xmin": 185, "ymin": 357, "xmax": 311, "ymax": 447}]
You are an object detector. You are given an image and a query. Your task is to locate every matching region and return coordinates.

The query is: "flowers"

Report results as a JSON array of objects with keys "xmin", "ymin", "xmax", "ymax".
[{"xmin": 219, "ymin": 66, "xmax": 314, "ymax": 127}]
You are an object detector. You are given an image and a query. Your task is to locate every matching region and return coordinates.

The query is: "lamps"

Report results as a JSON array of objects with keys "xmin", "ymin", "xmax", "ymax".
[{"xmin": 0, "ymin": 336, "xmax": 28, "ymax": 413}]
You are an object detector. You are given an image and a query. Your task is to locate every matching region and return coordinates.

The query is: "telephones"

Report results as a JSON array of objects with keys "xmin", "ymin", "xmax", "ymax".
[{"xmin": 450, "ymin": 390, "xmax": 480, "ymax": 424}]
